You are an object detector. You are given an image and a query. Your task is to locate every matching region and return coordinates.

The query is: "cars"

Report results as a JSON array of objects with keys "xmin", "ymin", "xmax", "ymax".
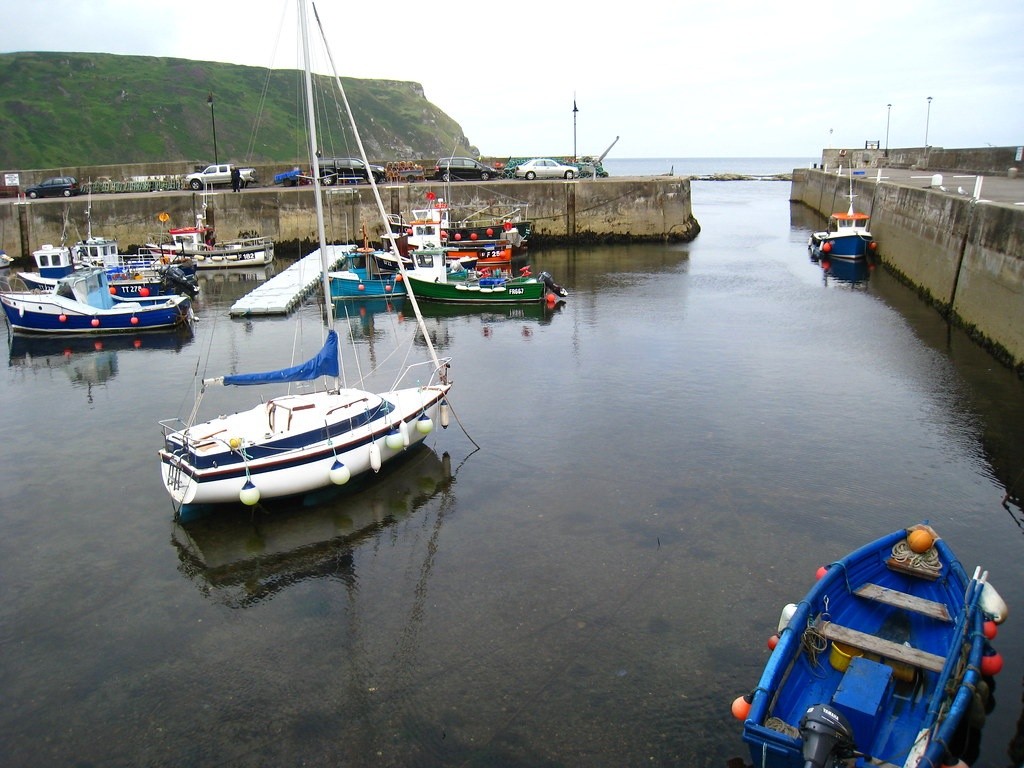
[
  {"xmin": 26, "ymin": 176, "xmax": 80, "ymax": 199},
  {"xmin": 515, "ymin": 158, "xmax": 580, "ymax": 179},
  {"xmin": 434, "ymin": 156, "xmax": 497, "ymax": 182}
]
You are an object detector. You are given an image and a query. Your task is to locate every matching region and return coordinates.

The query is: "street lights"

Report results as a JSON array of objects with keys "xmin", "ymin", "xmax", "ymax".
[{"xmin": 206, "ymin": 90, "xmax": 218, "ymax": 165}]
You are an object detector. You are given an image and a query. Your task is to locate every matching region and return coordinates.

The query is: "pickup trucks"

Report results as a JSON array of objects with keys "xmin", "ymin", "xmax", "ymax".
[{"xmin": 185, "ymin": 163, "xmax": 257, "ymax": 190}]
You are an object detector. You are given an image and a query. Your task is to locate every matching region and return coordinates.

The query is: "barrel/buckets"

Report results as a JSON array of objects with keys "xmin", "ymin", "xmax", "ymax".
[{"xmin": 830, "ymin": 641, "xmax": 864, "ymax": 673}]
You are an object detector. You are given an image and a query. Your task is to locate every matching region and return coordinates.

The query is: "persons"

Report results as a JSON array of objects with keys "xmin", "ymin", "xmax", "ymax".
[{"xmin": 231, "ymin": 166, "xmax": 240, "ymax": 193}]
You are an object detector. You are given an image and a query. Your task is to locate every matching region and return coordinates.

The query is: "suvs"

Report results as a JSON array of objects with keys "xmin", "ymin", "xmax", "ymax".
[{"xmin": 311, "ymin": 157, "xmax": 385, "ymax": 186}]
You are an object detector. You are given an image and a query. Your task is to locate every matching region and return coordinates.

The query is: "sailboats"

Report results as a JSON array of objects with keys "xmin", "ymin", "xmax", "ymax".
[{"xmin": 156, "ymin": 0, "xmax": 455, "ymax": 525}]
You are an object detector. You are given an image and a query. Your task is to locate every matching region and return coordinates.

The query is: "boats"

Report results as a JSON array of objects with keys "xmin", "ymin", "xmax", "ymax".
[
  {"xmin": 822, "ymin": 252, "xmax": 876, "ymax": 294},
  {"xmin": 731, "ymin": 519, "xmax": 1008, "ymax": 768},
  {"xmin": 808, "ymin": 157, "xmax": 878, "ymax": 259},
  {"xmin": 319, "ymin": 183, "xmax": 567, "ymax": 303},
  {"xmin": 0, "ymin": 175, "xmax": 274, "ymax": 355}
]
